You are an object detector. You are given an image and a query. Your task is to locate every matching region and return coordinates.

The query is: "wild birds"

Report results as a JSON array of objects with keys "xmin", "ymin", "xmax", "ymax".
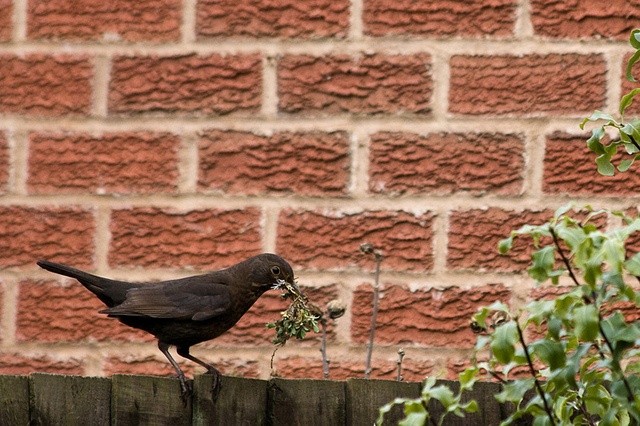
[{"xmin": 35, "ymin": 252, "xmax": 304, "ymax": 394}]
